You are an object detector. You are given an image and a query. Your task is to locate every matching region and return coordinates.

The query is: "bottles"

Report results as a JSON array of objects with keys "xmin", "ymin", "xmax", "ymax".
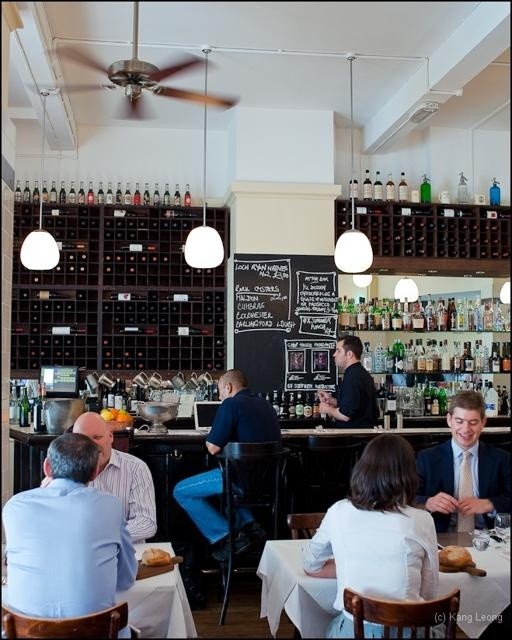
[
  {"xmin": 252, "ymin": 390, "xmax": 320, "ymax": 419},
  {"xmin": 457, "ymin": 171, "xmax": 469, "ymax": 204},
  {"xmin": 348, "ymin": 169, "xmax": 408, "ymax": 202},
  {"xmin": 14, "ymin": 179, "xmax": 192, "ymax": 208},
  {"xmin": 421, "ymin": 174, "xmax": 433, "ymax": 205},
  {"xmin": 102, "ymin": 378, "xmax": 139, "ymax": 412},
  {"xmin": 490, "ymin": 174, "xmax": 501, "ymax": 204},
  {"xmin": 337, "ymin": 205, "xmax": 510, "ymax": 258},
  {"xmin": 339, "ymin": 288, "xmax": 511, "ymax": 421},
  {"xmin": 8, "ymin": 385, "xmax": 48, "ymax": 435},
  {"xmin": 12, "ymin": 208, "xmax": 229, "ymax": 370}
]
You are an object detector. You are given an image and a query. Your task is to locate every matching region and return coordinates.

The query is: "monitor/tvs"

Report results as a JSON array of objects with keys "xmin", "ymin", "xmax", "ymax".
[
  {"xmin": 38, "ymin": 365, "xmax": 79, "ymax": 398},
  {"xmin": 194, "ymin": 401, "xmax": 223, "ymax": 435}
]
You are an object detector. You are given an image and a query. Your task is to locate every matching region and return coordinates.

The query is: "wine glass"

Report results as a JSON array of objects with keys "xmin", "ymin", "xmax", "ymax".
[{"xmin": 494, "ymin": 512, "xmax": 511, "ymax": 551}]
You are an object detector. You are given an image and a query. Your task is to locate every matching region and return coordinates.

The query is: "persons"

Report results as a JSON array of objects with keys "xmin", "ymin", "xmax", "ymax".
[
  {"xmin": 1, "ymin": 429, "xmax": 140, "ymax": 640},
  {"xmin": 73, "ymin": 409, "xmax": 160, "ymax": 546},
  {"xmin": 168, "ymin": 366, "xmax": 285, "ymax": 562},
  {"xmin": 300, "ymin": 432, "xmax": 441, "ymax": 639},
  {"xmin": 313, "ymin": 333, "xmax": 381, "ymax": 427},
  {"xmin": 411, "ymin": 387, "xmax": 511, "ymax": 532}
]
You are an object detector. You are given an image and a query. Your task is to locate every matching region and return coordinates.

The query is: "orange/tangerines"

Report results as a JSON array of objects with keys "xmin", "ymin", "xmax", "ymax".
[{"xmin": 100, "ymin": 408, "xmax": 131, "ymax": 423}]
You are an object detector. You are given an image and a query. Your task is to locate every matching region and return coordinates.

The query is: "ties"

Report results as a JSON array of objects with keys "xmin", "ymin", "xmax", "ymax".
[{"xmin": 457, "ymin": 452, "xmax": 475, "ymax": 533}]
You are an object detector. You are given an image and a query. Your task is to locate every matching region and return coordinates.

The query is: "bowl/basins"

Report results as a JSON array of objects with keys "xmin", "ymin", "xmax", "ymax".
[{"xmin": 135, "ymin": 401, "xmax": 182, "ymax": 433}]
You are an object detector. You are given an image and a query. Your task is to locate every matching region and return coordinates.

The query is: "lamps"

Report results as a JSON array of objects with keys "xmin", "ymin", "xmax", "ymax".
[
  {"xmin": 20, "ymin": 90, "xmax": 60, "ymax": 271},
  {"xmin": 185, "ymin": 42, "xmax": 226, "ymax": 271},
  {"xmin": 333, "ymin": 53, "xmax": 375, "ymax": 274}
]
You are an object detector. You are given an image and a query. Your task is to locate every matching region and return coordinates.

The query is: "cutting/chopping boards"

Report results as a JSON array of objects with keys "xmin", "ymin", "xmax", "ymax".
[
  {"xmin": 438, "ymin": 562, "xmax": 487, "ymax": 578},
  {"xmin": 136, "ymin": 556, "xmax": 183, "ymax": 580}
]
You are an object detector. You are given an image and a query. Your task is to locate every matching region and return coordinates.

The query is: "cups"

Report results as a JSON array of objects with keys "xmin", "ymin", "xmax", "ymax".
[
  {"xmin": 471, "ymin": 529, "xmax": 491, "ymax": 554},
  {"xmin": 473, "ymin": 193, "xmax": 488, "ymax": 206},
  {"xmin": 438, "ymin": 190, "xmax": 452, "ymax": 206},
  {"xmin": 83, "ymin": 373, "xmax": 213, "ymax": 390},
  {"xmin": 409, "ymin": 190, "xmax": 421, "ymax": 203}
]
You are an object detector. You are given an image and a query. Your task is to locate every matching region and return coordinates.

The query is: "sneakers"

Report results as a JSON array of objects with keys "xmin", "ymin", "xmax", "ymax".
[{"xmin": 212, "ymin": 532, "xmax": 250, "ymax": 561}]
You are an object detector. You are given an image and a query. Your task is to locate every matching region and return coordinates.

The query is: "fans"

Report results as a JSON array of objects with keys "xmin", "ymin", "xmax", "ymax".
[{"xmin": 36, "ymin": 2, "xmax": 240, "ymax": 124}]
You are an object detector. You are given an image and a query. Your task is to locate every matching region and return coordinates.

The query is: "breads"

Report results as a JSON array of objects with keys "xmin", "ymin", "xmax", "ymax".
[
  {"xmin": 438, "ymin": 544, "xmax": 473, "ymax": 567},
  {"xmin": 140, "ymin": 548, "xmax": 171, "ymax": 566}
]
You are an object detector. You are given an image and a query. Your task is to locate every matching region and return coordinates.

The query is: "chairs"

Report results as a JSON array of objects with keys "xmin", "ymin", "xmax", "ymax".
[
  {"xmin": 201, "ymin": 442, "xmax": 306, "ymax": 622},
  {"xmin": 342, "ymin": 585, "xmax": 461, "ymax": 640},
  {"xmin": 0, "ymin": 604, "xmax": 133, "ymax": 640},
  {"xmin": 284, "ymin": 508, "xmax": 328, "ymax": 539}
]
